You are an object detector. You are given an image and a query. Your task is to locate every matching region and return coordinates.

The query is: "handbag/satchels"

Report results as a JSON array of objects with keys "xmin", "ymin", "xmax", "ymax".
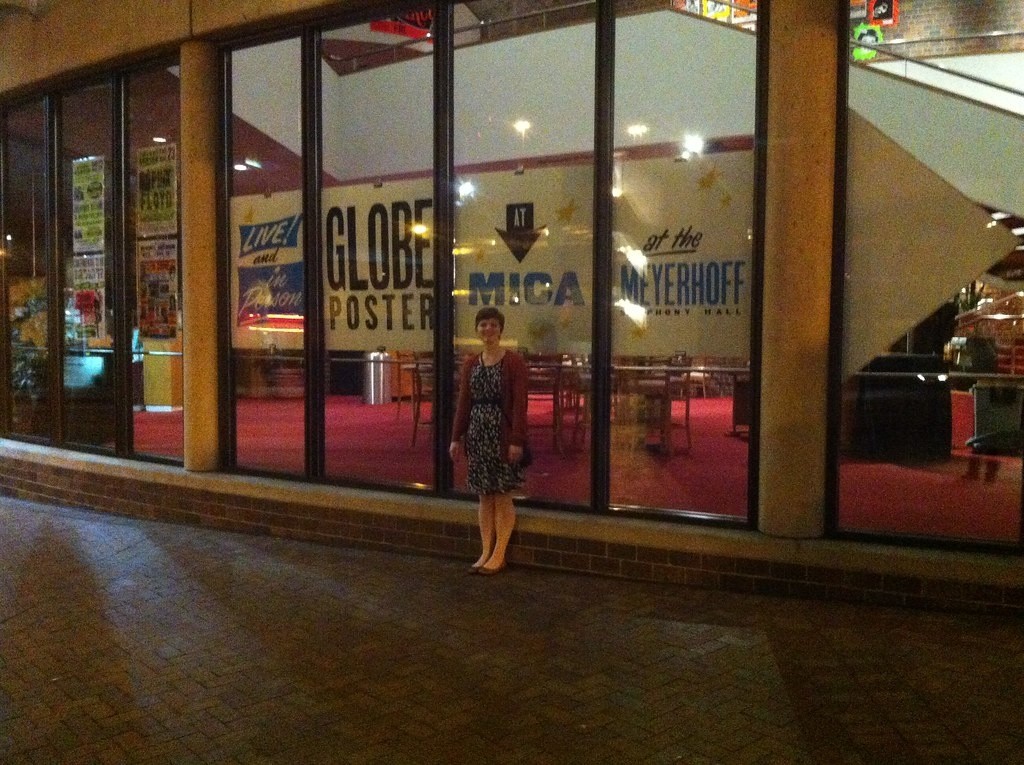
[{"xmin": 518, "ymin": 444, "xmax": 533, "ymax": 468}]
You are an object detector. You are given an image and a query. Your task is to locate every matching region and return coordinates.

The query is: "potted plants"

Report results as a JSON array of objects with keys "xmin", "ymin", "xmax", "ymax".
[{"xmin": 943, "ymin": 279, "xmax": 997, "ymax": 391}]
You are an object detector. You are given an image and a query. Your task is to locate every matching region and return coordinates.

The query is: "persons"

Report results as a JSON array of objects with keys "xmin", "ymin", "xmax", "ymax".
[{"xmin": 448, "ymin": 305, "xmax": 531, "ymax": 574}]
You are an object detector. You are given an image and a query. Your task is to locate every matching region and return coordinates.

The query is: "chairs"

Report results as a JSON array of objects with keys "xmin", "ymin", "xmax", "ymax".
[{"xmin": 389, "ymin": 350, "xmax": 750, "ymax": 462}]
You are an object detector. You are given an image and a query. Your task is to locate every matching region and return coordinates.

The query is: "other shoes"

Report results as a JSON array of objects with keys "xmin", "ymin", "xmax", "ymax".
[
  {"xmin": 479, "ymin": 557, "xmax": 507, "ymax": 575},
  {"xmin": 468, "ymin": 565, "xmax": 482, "ymax": 574}
]
